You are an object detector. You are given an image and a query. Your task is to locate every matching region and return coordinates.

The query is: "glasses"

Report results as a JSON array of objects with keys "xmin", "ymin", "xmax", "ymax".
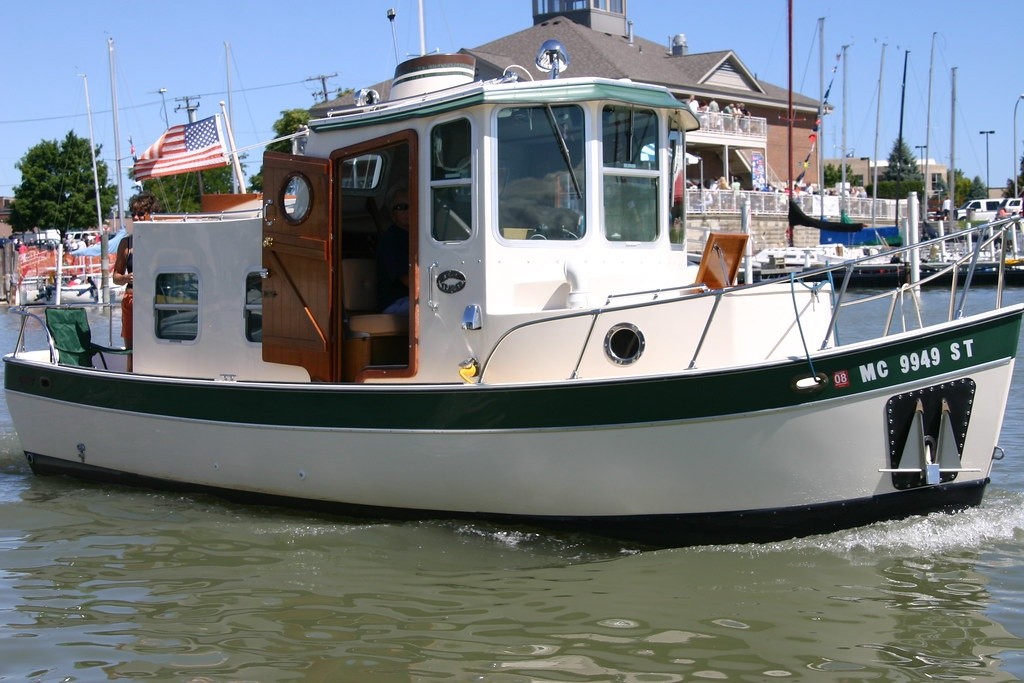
[{"xmin": 131, "ymin": 212, "xmax": 150, "ymax": 217}]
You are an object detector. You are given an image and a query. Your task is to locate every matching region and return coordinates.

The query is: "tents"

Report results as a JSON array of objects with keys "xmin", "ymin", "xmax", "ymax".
[{"xmin": 685, "ymin": 151, "xmax": 704, "ymax": 192}]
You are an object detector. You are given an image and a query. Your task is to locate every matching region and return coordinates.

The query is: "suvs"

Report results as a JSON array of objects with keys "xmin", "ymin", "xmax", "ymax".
[
  {"xmin": 997, "ymin": 197, "xmax": 1024, "ymax": 221},
  {"xmin": 954, "ymin": 198, "xmax": 1007, "ymax": 222},
  {"xmin": 62, "ymin": 231, "xmax": 100, "ymax": 246}
]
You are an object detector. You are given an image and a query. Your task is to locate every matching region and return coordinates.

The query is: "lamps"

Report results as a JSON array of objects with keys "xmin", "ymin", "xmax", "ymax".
[
  {"xmin": 354, "ymin": 88, "xmax": 380, "ymax": 108},
  {"xmin": 535, "ymin": 39, "xmax": 569, "ymax": 80}
]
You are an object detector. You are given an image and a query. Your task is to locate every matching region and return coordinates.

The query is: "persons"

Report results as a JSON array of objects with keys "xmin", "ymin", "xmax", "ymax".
[
  {"xmin": 373, "ymin": 180, "xmax": 410, "ymax": 313},
  {"xmin": 997, "ymin": 206, "xmax": 1006, "ymax": 218},
  {"xmin": 112, "ymin": 193, "xmax": 161, "ymax": 373},
  {"xmin": 935, "ymin": 195, "xmax": 958, "ymax": 221},
  {"xmin": 687, "ymin": 92, "xmax": 751, "ymax": 134},
  {"xmin": 14, "ymin": 233, "xmax": 110, "ymax": 262},
  {"xmin": 929, "ymin": 244, "xmax": 938, "ymax": 262},
  {"xmin": 685, "ymin": 175, "xmax": 868, "ymax": 216},
  {"xmin": 890, "ymin": 253, "xmax": 903, "ymax": 263}
]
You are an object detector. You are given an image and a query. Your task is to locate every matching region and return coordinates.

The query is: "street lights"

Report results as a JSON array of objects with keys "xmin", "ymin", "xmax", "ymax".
[
  {"xmin": 915, "ymin": 144, "xmax": 927, "ymax": 177},
  {"xmin": 859, "ymin": 157, "xmax": 871, "ymax": 187},
  {"xmin": 977, "ymin": 129, "xmax": 996, "ymax": 198},
  {"xmin": 1013, "ymin": 94, "xmax": 1024, "ymax": 199}
]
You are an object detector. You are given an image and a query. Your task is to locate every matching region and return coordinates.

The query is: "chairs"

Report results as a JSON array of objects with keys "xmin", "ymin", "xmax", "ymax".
[
  {"xmin": 341, "ymin": 253, "xmax": 409, "ymax": 337},
  {"xmin": 46, "ymin": 307, "xmax": 133, "ymax": 370}
]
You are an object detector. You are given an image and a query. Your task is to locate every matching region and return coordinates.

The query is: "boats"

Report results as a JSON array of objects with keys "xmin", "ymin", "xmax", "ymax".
[{"xmin": 4, "ymin": 0, "xmax": 1024, "ymax": 549}]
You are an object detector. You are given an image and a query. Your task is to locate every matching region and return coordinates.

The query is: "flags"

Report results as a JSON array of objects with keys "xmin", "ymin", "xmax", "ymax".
[{"xmin": 133, "ymin": 113, "xmax": 232, "ymax": 182}]
